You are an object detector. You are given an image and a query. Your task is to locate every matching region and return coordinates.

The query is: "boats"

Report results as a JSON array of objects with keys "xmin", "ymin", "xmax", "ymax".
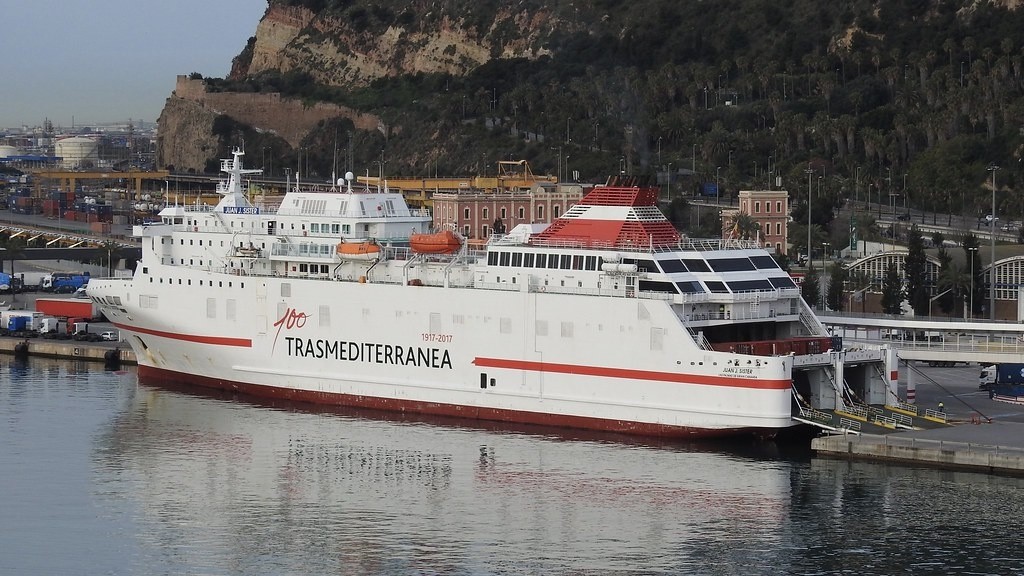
[
  {"xmin": 85, "ymin": 146, "xmax": 956, "ymax": 442},
  {"xmin": 409, "ymin": 231, "xmax": 463, "ymax": 254},
  {"xmin": 336, "ymin": 240, "xmax": 383, "ymax": 262}
]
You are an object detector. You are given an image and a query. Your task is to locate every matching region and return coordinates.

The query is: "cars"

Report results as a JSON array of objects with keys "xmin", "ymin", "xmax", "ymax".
[
  {"xmin": 100, "ymin": 331, "xmax": 118, "ymax": 341},
  {"xmin": 986, "ymin": 215, "xmax": 999, "ymax": 222},
  {"xmin": 77, "ymin": 284, "xmax": 88, "ymax": 293},
  {"xmin": 700, "ymin": 184, "xmax": 722, "ymax": 197},
  {"xmin": 54, "ymin": 285, "xmax": 76, "ymax": 294},
  {"xmin": 58, "ymin": 317, "xmax": 67, "ymax": 322}
]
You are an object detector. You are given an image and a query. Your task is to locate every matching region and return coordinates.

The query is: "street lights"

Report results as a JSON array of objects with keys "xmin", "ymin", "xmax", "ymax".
[
  {"xmin": 9, "ymin": 255, "xmax": 16, "ymax": 300},
  {"xmin": 489, "ymin": 88, "xmax": 1000, "ymax": 342}
]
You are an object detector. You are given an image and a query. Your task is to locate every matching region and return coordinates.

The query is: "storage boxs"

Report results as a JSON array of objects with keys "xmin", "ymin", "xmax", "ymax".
[{"xmin": 712, "ymin": 336, "xmax": 843, "ymax": 356}]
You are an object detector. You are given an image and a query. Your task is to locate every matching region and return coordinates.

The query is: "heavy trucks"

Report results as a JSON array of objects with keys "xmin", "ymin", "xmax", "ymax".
[
  {"xmin": 7, "ymin": 317, "xmax": 39, "ymax": 338},
  {"xmin": 40, "ymin": 318, "xmax": 71, "ymax": 340},
  {"xmin": 72, "ymin": 323, "xmax": 102, "ymax": 342}
]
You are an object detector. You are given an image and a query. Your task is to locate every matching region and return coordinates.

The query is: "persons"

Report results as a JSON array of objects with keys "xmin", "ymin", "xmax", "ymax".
[{"xmin": 938, "ymin": 402, "xmax": 943, "ymax": 412}]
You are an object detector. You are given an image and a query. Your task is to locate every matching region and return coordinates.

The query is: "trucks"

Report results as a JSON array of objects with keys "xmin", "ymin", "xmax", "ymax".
[
  {"xmin": 0, "ymin": 272, "xmax": 25, "ymax": 294},
  {"xmin": 40, "ymin": 272, "xmax": 90, "ymax": 288},
  {"xmin": 979, "ymin": 363, "xmax": 1024, "ymax": 391}
]
